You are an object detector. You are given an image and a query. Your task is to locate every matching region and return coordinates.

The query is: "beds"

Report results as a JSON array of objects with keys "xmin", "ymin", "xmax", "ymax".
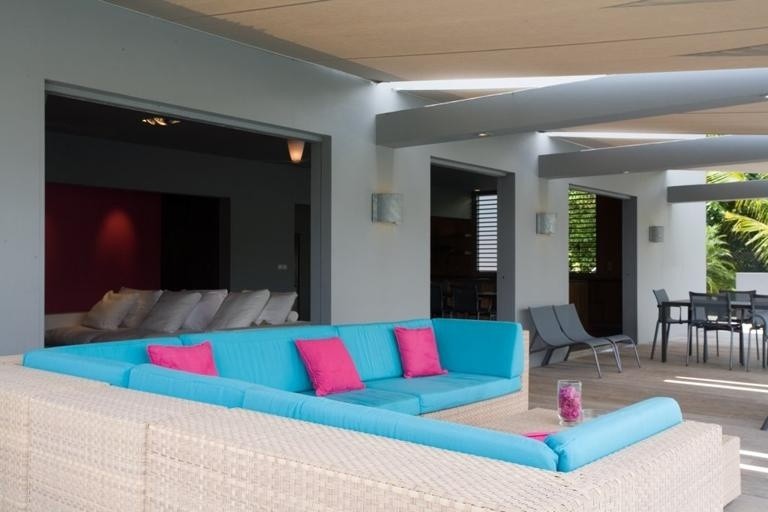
[{"xmin": 44, "ymin": 311, "xmax": 299, "ymax": 348}]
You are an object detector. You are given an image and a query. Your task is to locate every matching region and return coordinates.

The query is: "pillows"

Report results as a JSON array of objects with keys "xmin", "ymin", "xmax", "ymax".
[
  {"xmin": 393, "ymin": 327, "xmax": 448, "ymax": 378},
  {"xmin": 81, "ymin": 286, "xmax": 297, "ymax": 335},
  {"xmin": 294, "ymin": 336, "xmax": 365, "ymax": 397},
  {"xmin": 146, "ymin": 342, "xmax": 218, "ymax": 376}
]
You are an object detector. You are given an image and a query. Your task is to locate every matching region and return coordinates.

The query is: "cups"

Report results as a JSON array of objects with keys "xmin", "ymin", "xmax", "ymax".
[{"xmin": 557, "ymin": 379, "xmax": 583, "ymax": 426}]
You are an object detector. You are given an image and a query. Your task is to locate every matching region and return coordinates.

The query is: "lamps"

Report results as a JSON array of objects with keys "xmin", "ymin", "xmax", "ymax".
[
  {"xmin": 536, "ymin": 211, "xmax": 556, "ymax": 235},
  {"xmin": 372, "ymin": 192, "xmax": 402, "ymax": 224},
  {"xmin": 287, "ymin": 140, "xmax": 305, "ymax": 163},
  {"xmin": 649, "ymin": 226, "xmax": 664, "ymax": 243}
]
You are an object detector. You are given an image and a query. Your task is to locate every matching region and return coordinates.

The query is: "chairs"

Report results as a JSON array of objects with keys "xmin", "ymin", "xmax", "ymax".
[
  {"xmin": 430, "ymin": 283, "xmax": 447, "ymax": 318},
  {"xmin": 528, "ymin": 306, "xmax": 622, "ymax": 378},
  {"xmin": 650, "ymin": 288, "xmax": 768, "ymax": 372},
  {"xmin": 545, "ymin": 304, "xmax": 641, "ymax": 368},
  {"xmin": 450, "ymin": 284, "xmax": 496, "ymax": 320}
]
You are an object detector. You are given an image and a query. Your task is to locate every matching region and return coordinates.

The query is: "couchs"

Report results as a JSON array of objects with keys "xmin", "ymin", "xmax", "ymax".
[{"xmin": 0, "ymin": 318, "xmax": 741, "ymax": 512}]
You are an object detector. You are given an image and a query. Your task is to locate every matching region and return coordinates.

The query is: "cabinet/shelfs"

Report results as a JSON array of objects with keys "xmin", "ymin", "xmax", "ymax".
[
  {"xmin": 478, "ymin": 278, "xmax": 496, "ymax": 309},
  {"xmin": 570, "ymin": 279, "xmax": 605, "ymax": 319}
]
